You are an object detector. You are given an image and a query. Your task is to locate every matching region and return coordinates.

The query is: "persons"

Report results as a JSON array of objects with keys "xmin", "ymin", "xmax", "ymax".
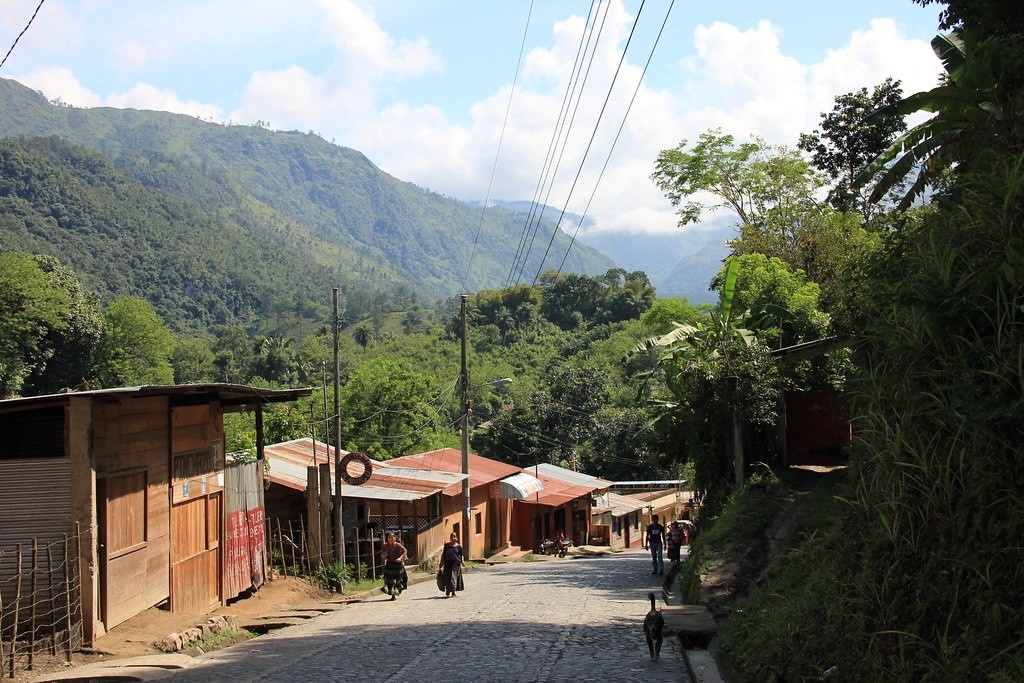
[
  {"xmin": 645, "ymin": 514, "xmax": 666, "ymax": 576},
  {"xmin": 439, "ymin": 532, "xmax": 465, "ymax": 596},
  {"xmin": 381, "ymin": 532, "xmax": 407, "ymax": 593},
  {"xmin": 667, "ymin": 521, "xmax": 684, "ymax": 562}
]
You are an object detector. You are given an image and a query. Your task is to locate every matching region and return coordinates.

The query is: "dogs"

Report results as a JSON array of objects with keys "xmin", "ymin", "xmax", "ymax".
[{"xmin": 644, "ymin": 593, "xmax": 665, "ymax": 663}]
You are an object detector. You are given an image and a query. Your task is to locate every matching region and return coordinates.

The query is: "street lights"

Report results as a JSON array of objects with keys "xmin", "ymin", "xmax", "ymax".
[{"xmin": 459, "ymin": 376, "xmax": 514, "ymax": 561}]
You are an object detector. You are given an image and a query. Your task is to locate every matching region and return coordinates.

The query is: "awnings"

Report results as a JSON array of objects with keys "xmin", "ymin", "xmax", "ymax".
[{"xmin": 499, "ymin": 473, "xmax": 543, "ymax": 498}]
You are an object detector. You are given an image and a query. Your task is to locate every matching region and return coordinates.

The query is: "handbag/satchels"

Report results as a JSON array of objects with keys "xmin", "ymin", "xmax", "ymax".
[{"xmin": 437, "ymin": 567, "xmax": 445, "ymax": 592}]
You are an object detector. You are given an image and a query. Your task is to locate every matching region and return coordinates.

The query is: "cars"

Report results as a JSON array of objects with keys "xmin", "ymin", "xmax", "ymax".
[{"xmin": 670, "ymin": 519, "xmax": 695, "ymax": 538}]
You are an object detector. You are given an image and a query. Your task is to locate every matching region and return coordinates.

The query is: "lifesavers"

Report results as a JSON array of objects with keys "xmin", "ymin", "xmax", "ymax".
[{"xmin": 339, "ymin": 452, "xmax": 373, "ymax": 486}]
